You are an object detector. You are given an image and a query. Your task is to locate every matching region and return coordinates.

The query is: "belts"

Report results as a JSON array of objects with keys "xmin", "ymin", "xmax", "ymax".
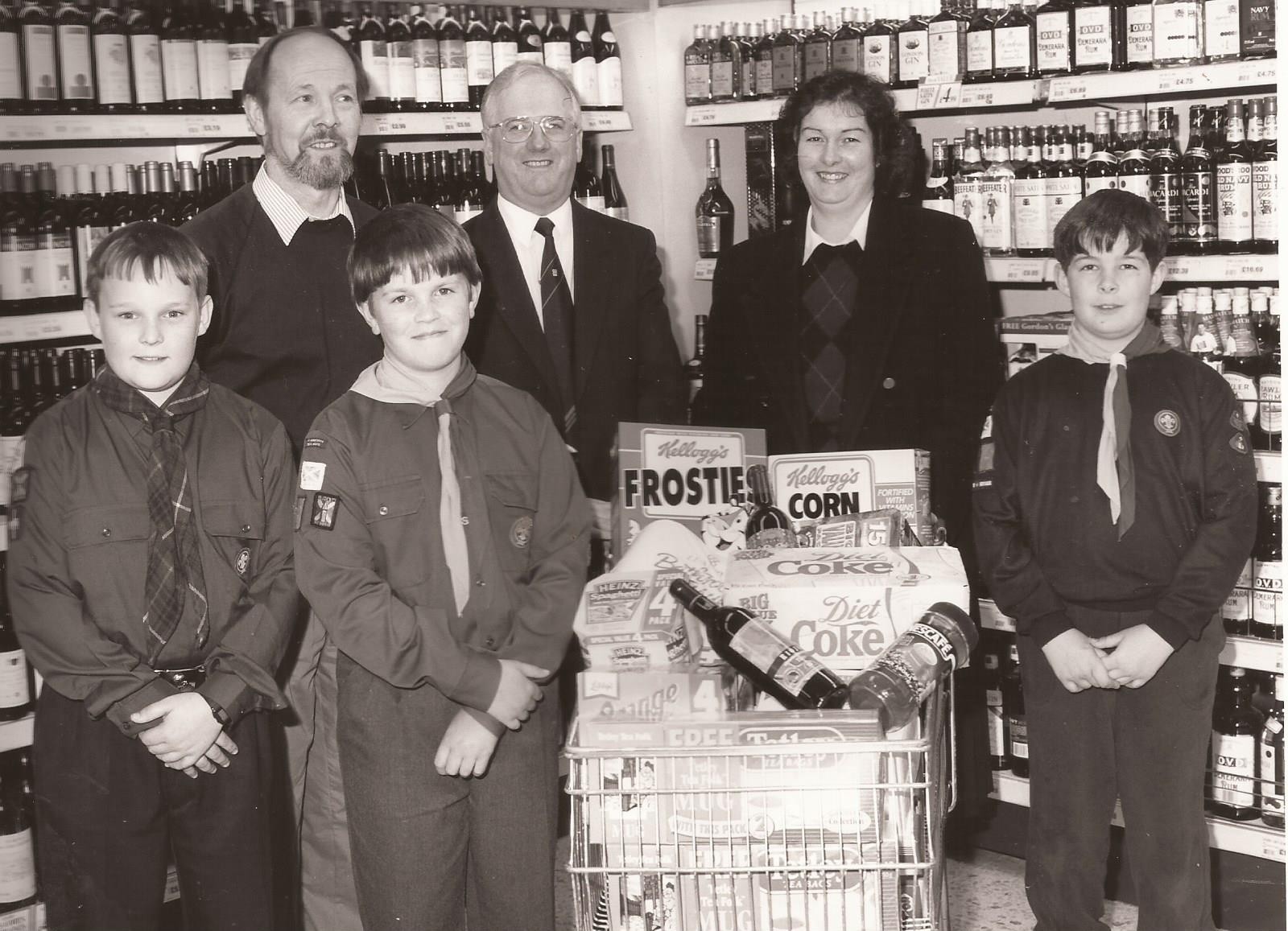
[{"xmin": 159, "ymin": 669, "xmax": 204, "ymax": 693}]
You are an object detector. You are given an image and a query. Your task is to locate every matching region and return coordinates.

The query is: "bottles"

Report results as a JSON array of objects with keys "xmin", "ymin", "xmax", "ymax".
[
  {"xmin": 754, "ymin": 19, "xmax": 783, "ymax": 99},
  {"xmin": 0, "ymin": 349, "xmax": 98, "ymax": 721},
  {"xmin": 683, "ymin": 24, "xmax": 718, "ymax": 106},
  {"xmin": 895, "ymin": 0, "xmax": 978, "ymax": 90},
  {"xmin": 0, "ymin": 148, "xmax": 498, "ymax": 315},
  {"xmin": 0, "ymin": 1, "xmax": 623, "ymax": 115},
  {"xmin": 983, "ymin": 123, "xmax": 1094, "ymax": 258},
  {"xmin": 1209, "ymin": 96, "xmax": 1278, "ymax": 255},
  {"xmin": 1081, "ymin": 109, "xmax": 1150, "ymax": 200},
  {"xmin": 1146, "ymin": 286, "xmax": 1280, "ymax": 452},
  {"xmin": 1150, "ymin": 0, "xmax": 1278, "ymax": 69},
  {"xmin": 1034, "ymin": 0, "xmax": 1152, "ymax": 78},
  {"xmin": 983, "ymin": 642, "xmax": 1030, "ymax": 778},
  {"xmin": 711, "ymin": 21, "xmax": 759, "ymax": 104},
  {"xmin": 965, "ymin": 0, "xmax": 1037, "ymax": 84},
  {"xmin": 849, "ymin": 601, "xmax": 980, "ymax": 733},
  {"xmin": 684, "ymin": 315, "xmax": 706, "ymax": 428},
  {"xmin": 0, "ymin": 747, "xmax": 37, "ymax": 912},
  {"xmin": 1217, "ymin": 486, "xmax": 1282, "ymax": 640},
  {"xmin": 1202, "ymin": 666, "xmax": 1286, "ymax": 828},
  {"xmin": 694, "ymin": 139, "xmax": 734, "ymax": 258},
  {"xmin": 772, "ymin": 10, "xmax": 842, "ymax": 99},
  {"xmin": 921, "ymin": 127, "xmax": 988, "ymax": 254},
  {"xmin": 572, "ymin": 145, "xmax": 628, "ymax": 220},
  {"xmin": 830, "ymin": 7, "xmax": 909, "ymax": 90},
  {"xmin": 745, "ymin": 464, "xmax": 797, "ymax": 547},
  {"xmin": 1145, "ymin": 104, "xmax": 1219, "ymax": 256},
  {"xmin": 670, "ymin": 578, "xmax": 850, "ymax": 710}
]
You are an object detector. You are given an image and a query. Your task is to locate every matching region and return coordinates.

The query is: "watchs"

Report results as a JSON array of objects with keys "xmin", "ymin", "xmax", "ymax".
[{"xmin": 201, "ymin": 694, "xmax": 231, "ymax": 725}]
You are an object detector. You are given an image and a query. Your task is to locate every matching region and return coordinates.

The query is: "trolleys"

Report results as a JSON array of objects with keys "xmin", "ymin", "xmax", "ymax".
[{"xmin": 569, "ymin": 680, "xmax": 965, "ymax": 931}]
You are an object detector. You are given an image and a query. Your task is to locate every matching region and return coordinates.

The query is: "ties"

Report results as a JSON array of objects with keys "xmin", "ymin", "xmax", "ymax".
[
  {"xmin": 535, "ymin": 216, "xmax": 579, "ymax": 448},
  {"xmin": 1051, "ymin": 313, "xmax": 1176, "ymax": 541},
  {"xmin": 94, "ymin": 355, "xmax": 212, "ymax": 664},
  {"xmin": 347, "ymin": 349, "xmax": 477, "ymax": 618}
]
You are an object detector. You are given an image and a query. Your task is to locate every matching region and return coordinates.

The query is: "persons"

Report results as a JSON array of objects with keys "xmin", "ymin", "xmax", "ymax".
[
  {"xmin": 174, "ymin": 27, "xmax": 385, "ymax": 931},
  {"xmin": 454, "ymin": 61, "xmax": 686, "ymax": 587},
  {"xmin": 292, "ymin": 202, "xmax": 592, "ymax": 931},
  {"xmin": 6, "ymin": 220, "xmax": 299, "ymax": 931},
  {"xmin": 971, "ymin": 188, "xmax": 1258, "ymax": 931},
  {"xmin": 691, "ymin": 70, "xmax": 1004, "ymax": 858}
]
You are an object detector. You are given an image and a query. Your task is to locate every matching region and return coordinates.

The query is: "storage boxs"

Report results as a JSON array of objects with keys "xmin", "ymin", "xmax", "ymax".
[{"xmin": 573, "ymin": 422, "xmax": 970, "ymax": 931}]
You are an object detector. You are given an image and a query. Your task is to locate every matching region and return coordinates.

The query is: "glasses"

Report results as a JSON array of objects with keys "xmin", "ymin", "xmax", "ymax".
[{"xmin": 486, "ymin": 115, "xmax": 581, "ymax": 143}]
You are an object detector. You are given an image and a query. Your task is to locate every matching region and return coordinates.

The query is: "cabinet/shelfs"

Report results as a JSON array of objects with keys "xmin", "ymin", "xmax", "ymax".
[
  {"xmin": 685, "ymin": 54, "xmax": 1282, "ymax": 865},
  {"xmin": 0, "ymin": 111, "xmax": 633, "ymax": 931}
]
